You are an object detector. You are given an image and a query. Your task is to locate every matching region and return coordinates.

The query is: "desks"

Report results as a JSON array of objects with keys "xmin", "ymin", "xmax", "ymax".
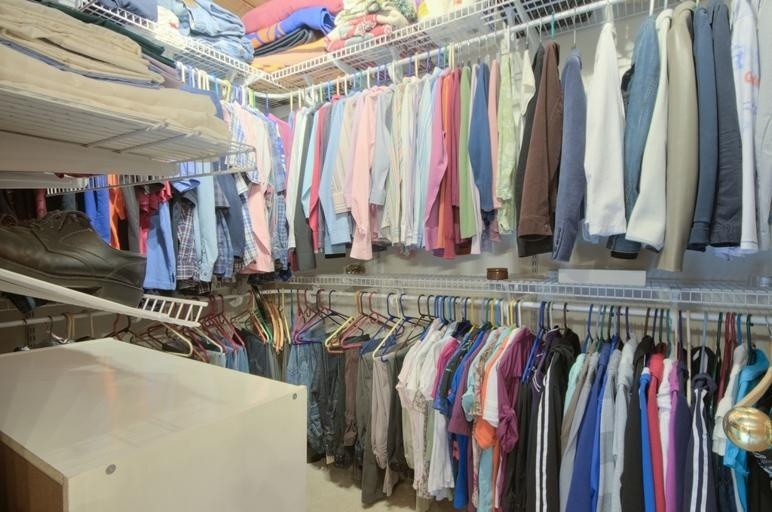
[{"xmin": 0, "ymin": 341, "xmax": 311, "ymax": 510}]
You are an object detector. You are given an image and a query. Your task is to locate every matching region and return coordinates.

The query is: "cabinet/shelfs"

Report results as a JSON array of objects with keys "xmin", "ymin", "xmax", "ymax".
[
  {"xmin": 0, "ymin": 2, "xmax": 272, "ymax": 333},
  {"xmin": 272, "ymin": 0, "xmax": 771, "ymax": 310}
]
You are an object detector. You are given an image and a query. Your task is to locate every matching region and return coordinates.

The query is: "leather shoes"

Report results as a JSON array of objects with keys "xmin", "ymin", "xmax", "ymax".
[{"xmin": 1, "ymin": 208, "xmax": 148, "ymax": 309}]
